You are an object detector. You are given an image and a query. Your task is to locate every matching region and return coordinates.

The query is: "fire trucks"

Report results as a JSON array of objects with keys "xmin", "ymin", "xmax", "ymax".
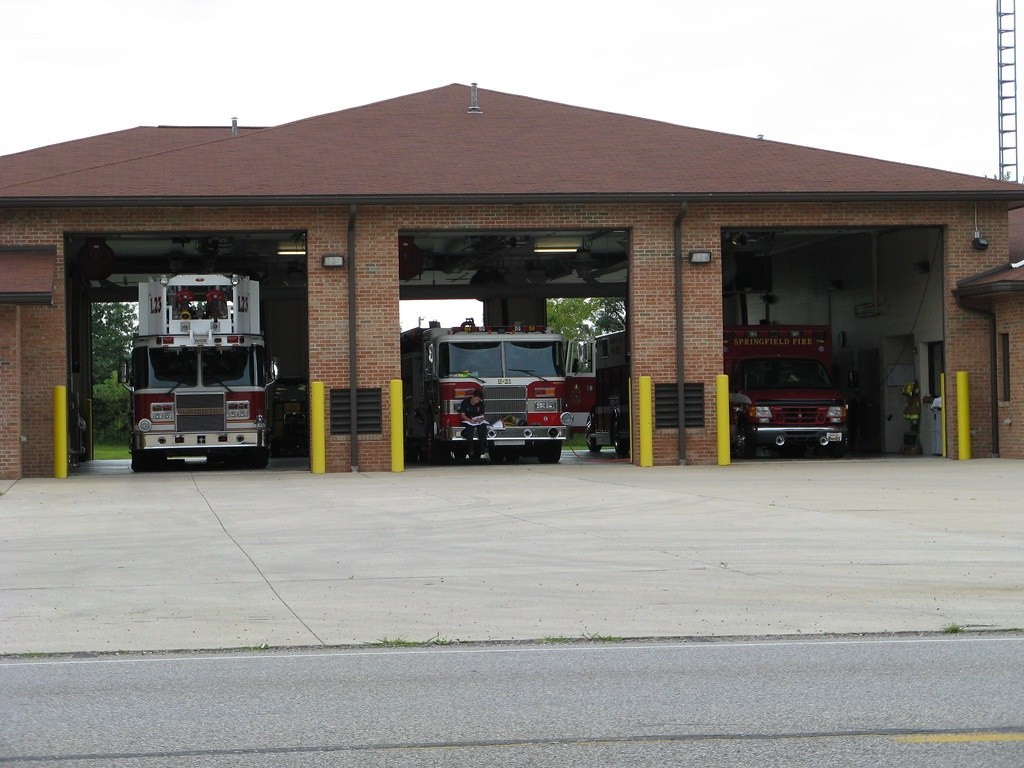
[
  {"xmin": 586, "ymin": 323, "xmax": 858, "ymax": 463},
  {"xmin": 113, "ymin": 269, "xmax": 284, "ymax": 473},
  {"xmin": 399, "ymin": 316, "xmax": 598, "ymax": 467}
]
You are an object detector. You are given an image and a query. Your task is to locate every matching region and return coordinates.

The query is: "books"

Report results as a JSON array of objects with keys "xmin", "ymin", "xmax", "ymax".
[{"xmin": 462, "ymin": 416, "xmax": 507, "ymax": 431}]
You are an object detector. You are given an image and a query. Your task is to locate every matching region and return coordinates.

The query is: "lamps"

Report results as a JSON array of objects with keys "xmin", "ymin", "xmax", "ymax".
[
  {"xmin": 322, "ymin": 255, "xmax": 345, "ymax": 268},
  {"xmin": 533, "ymin": 236, "xmax": 584, "ymax": 253},
  {"xmin": 972, "ymin": 238, "xmax": 989, "ymax": 251},
  {"xmin": 688, "ymin": 251, "xmax": 711, "ymax": 264}
]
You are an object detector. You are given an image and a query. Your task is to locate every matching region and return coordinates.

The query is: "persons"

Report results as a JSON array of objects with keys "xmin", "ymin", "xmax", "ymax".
[{"xmin": 459, "ymin": 390, "xmax": 490, "ymax": 461}]
